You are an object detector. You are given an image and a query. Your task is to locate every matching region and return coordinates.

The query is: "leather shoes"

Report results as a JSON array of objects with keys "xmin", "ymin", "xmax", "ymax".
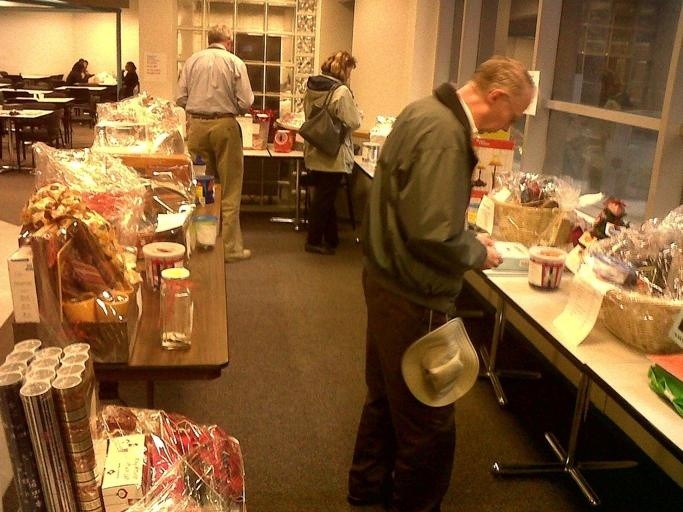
[{"xmin": 304, "ymin": 242, "xmax": 335, "ymax": 255}]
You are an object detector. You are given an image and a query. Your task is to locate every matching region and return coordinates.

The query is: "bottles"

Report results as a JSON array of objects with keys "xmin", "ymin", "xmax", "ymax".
[
  {"xmin": 159, "ymin": 267, "xmax": 195, "ymax": 350},
  {"xmin": 362, "ymin": 142, "xmax": 374, "ymax": 162},
  {"xmin": 368, "ymin": 144, "xmax": 379, "ymax": 168}
]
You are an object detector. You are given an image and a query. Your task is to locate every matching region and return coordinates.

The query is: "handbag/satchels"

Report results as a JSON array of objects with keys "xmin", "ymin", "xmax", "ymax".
[{"xmin": 298, "ymin": 83, "xmax": 354, "ymax": 157}]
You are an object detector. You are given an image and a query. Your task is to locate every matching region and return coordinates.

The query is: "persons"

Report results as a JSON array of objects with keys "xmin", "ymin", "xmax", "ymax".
[
  {"xmin": 346, "ymin": 48, "xmax": 535, "ymax": 511},
  {"xmin": 67, "ymin": 58, "xmax": 95, "ymax": 104},
  {"xmin": 173, "ymin": 23, "xmax": 255, "ymax": 264},
  {"xmin": 116, "ymin": 61, "xmax": 138, "ymax": 99},
  {"xmin": 300, "ymin": 48, "xmax": 364, "ymax": 256}
]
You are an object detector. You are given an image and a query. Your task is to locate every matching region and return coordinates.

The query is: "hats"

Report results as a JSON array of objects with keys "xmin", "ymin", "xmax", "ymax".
[{"xmin": 400, "ymin": 316, "xmax": 480, "ymax": 407}]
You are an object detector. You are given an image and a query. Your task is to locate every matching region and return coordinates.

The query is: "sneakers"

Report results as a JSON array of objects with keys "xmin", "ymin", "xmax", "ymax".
[{"xmin": 224, "ymin": 249, "xmax": 251, "ymax": 262}]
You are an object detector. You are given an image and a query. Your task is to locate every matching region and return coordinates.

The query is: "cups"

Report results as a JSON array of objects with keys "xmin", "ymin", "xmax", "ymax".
[
  {"xmin": 63, "ymin": 290, "xmax": 96, "ymax": 322},
  {"xmin": 97, "ymin": 292, "xmax": 129, "ymax": 324}
]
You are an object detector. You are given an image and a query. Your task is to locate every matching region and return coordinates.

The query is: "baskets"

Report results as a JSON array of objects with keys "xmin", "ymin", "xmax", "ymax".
[
  {"xmin": 488, "ymin": 185, "xmax": 574, "ymax": 248},
  {"xmin": 599, "ymin": 290, "xmax": 681, "ymax": 355}
]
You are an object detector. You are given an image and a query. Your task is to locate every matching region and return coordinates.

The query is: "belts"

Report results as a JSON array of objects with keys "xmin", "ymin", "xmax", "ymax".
[{"xmin": 189, "ymin": 113, "xmax": 235, "ymax": 120}]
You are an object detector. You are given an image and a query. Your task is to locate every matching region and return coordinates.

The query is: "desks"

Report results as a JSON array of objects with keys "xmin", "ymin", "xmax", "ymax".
[
  {"xmin": 93, "ymin": 181, "xmax": 230, "ymax": 410},
  {"xmin": 0, "ymin": 83, "xmax": 113, "ymax": 173}
]
